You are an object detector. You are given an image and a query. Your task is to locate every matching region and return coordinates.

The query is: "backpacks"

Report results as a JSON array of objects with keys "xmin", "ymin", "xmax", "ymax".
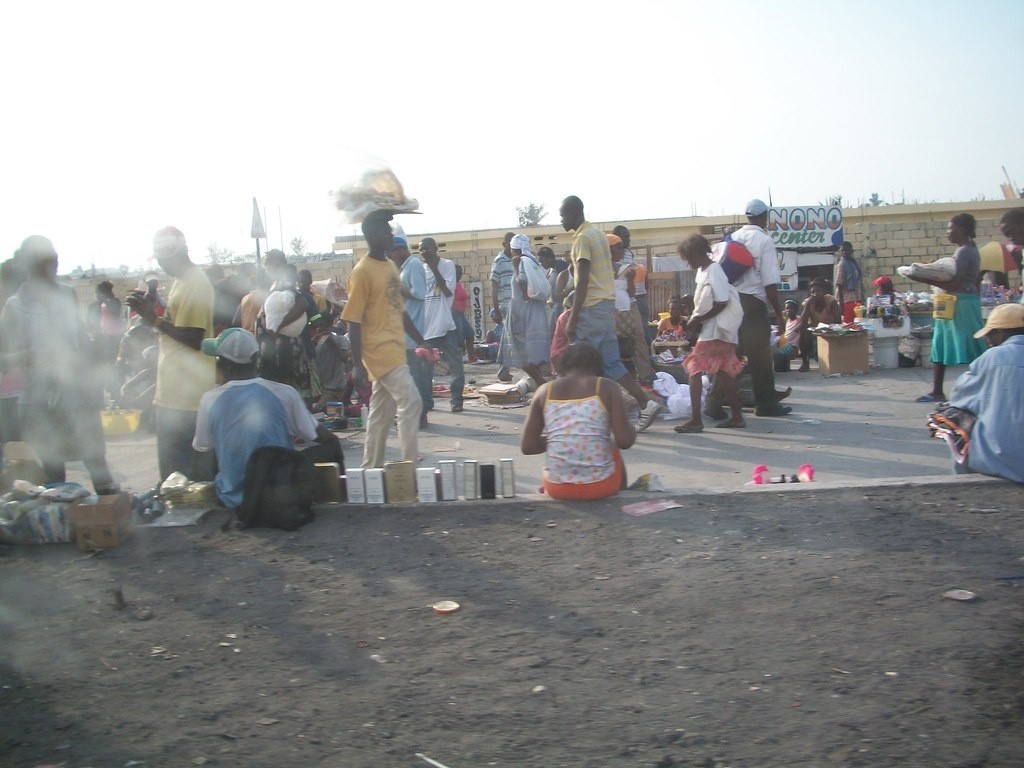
[
  {"xmin": 718, "ymin": 228, "xmax": 753, "ymax": 282},
  {"xmin": 235, "ymin": 444, "xmax": 317, "ymax": 532}
]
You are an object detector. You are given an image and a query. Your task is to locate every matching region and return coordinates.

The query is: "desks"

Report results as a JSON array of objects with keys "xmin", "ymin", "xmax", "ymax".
[
  {"xmin": 812, "ymin": 332, "xmax": 870, "ymax": 376},
  {"xmin": 654, "ymin": 341, "xmax": 690, "ymax": 358}
]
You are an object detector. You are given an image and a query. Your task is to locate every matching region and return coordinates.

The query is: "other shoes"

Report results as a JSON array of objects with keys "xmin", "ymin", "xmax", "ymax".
[
  {"xmin": 777, "ymin": 387, "xmax": 791, "ymax": 401},
  {"xmin": 704, "ymin": 406, "xmax": 728, "ymax": 419},
  {"xmin": 800, "ymin": 362, "xmax": 809, "ymax": 372},
  {"xmin": 638, "ymin": 374, "xmax": 657, "ymax": 386},
  {"xmin": 420, "ymin": 415, "xmax": 427, "ymax": 426},
  {"xmin": 500, "ymin": 374, "xmax": 513, "ymax": 384},
  {"xmin": 451, "ymin": 402, "xmax": 462, "ymax": 412},
  {"xmin": 737, "ymin": 394, "xmax": 755, "ymax": 407},
  {"xmin": 470, "ymin": 359, "xmax": 484, "ymax": 364},
  {"xmin": 755, "ymin": 403, "xmax": 792, "ymax": 416}
]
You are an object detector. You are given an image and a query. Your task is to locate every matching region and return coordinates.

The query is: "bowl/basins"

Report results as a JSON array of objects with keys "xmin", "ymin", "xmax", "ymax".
[
  {"xmin": 100, "ymin": 409, "xmax": 143, "ymax": 436},
  {"xmin": 658, "ymin": 312, "xmax": 670, "ymax": 319}
]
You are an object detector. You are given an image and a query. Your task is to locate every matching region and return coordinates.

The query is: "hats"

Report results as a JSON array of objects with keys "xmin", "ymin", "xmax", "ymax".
[
  {"xmin": 143, "ymin": 269, "xmax": 159, "ymax": 282},
  {"xmin": 147, "ymin": 226, "xmax": 187, "ymax": 261},
  {"xmin": 745, "ymin": 199, "xmax": 770, "ymax": 217},
  {"xmin": 606, "ymin": 233, "xmax": 622, "ymax": 246},
  {"xmin": 201, "ymin": 327, "xmax": 260, "ymax": 364},
  {"xmin": 384, "ymin": 237, "xmax": 407, "ymax": 257},
  {"xmin": 510, "ymin": 233, "xmax": 530, "ymax": 250},
  {"xmin": 973, "ymin": 303, "xmax": 1024, "ymax": 339}
]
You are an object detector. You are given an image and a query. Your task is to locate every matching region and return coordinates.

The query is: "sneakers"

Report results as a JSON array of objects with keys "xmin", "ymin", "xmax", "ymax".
[{"xmin": 634, "ymin": 400, "xmax": 662, "ymax": 432}]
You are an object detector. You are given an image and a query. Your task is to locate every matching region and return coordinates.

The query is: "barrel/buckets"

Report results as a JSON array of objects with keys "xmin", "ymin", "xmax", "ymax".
[
  {"xmin": 919, "ymin": 339, "xmax": 934, "ymax": 369},
  {"xmin": 930, "ymin": 294, "xmax": 957, "ymax": 320},
  {"xmin": 326, "ymin": 402, "xmax": 343, "ymax": 417},
  {"xmin": 871, "ymin": 337, "xmax": 899, "ymax": 369}
]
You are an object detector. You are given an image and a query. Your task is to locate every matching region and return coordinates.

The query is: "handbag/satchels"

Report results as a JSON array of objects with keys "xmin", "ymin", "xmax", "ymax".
[{"xmin": 520, "ymin": 255, "xmax": 552, "ymax": 301}]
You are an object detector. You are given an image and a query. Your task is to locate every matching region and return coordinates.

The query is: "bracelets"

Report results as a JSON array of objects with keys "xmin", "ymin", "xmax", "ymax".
[{"xmin": 153, "ymin": 317, "xmax": 164, "ymax": 327}]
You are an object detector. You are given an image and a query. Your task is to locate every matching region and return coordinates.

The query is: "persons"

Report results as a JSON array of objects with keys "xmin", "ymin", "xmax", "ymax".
[
  {"xmin": 949, "ymin": 303, "xmax": 1024, "ymax": 483},
  {"xmin": 831, "ymin": 241, "xmax": 865, "ymax": 324},
  {"xmin": 0, "ymin": 168, "xmax": 843, "ymax": 530},
  {"xmin": 902, "ymin": 213, "xmax": 982, "ymax": 403},
  {"xmin": 190, "ymin": 327, "xmax": 346, "ymax": 513},
  {"xmin": 519, "ymin": 342, "xmax": 636, "ymax": 502},
  {"xmin": 997, "ymin": 211, "xmax": 1024, "ymax": 304}
]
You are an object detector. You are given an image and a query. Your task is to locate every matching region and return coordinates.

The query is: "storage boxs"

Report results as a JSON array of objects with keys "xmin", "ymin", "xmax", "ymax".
[
  {"xmin": 852, "ymin": 316, "xmax": 911, "ymax": 338},
  {"xmin": 75, "ymin": 491, "xmax": 133, "ymax": 550},
  {"xmin": 313, "ymin": 459, "xmax": 516, "ymax": 505}
]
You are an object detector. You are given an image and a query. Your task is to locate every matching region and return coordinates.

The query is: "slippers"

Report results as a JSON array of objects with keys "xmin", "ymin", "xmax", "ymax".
[
  {"xmin": 674, "ymin": 421, "xmax": 704, "ymax": 433},
  {"xmin": 916, "ymin": 393, "xmax": 946, "ymax": 402},
  {"xmin": 716, "ymin": 418, "xmax": 746, "ymax": 427}
]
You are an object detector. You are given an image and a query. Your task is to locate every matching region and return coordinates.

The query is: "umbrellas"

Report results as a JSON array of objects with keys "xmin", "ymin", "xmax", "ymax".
[{"xmin": 973, "ymin": 242, "xmax": 1024, "ymax": 274}]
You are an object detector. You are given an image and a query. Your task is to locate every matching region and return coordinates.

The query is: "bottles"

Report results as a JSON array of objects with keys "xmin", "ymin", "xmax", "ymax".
[
  {"xmin": 140, "ymin": 488, "xmax": 161, "ymax": 523},
  {"xmin": 360, "ymin": 403, "xmax": 369, "ymax": 428}
]
